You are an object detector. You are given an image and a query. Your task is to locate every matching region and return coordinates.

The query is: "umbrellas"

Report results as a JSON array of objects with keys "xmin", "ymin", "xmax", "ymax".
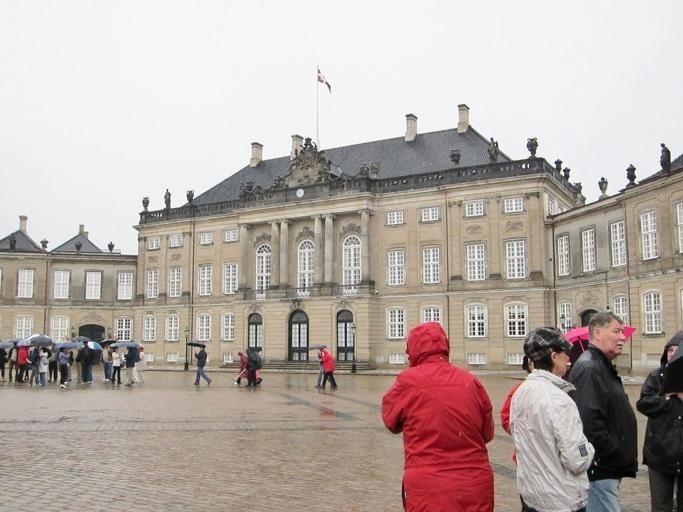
[
  {"xmin": 29, "ymin": 335, "xmax": 52, "ymax": 346},
  {"xmin": 82, "ymin": 340, "xmax": 102, "ymax": 351},
  {"xmin": 16, "ymin": 338, "xmax": 32, "ymax": 347},
  {"xmin": 98, "ymin": 338, "xmax": 117, "ymax": 349},
  {"xmin": 186, "ymin": 340, "xmax": 206, "ymax": 348},
  {"xmin": 51, "ymin": 343, "xmax": 62, "ymax": 352},
  {"xmin": 123, "ymin": 342, "xmax": 139, "ymax": 348},
  {"xmin": 110, "ymin": 342, "xmax": 124, "ymax": 347},
  {"xmin": 564, "ymin": 324, "xmax": 636, "ymax": 355},
  {"xmin": 8, "ymin": 339, "xmax": 20, "ymax": 343},
  {"xmin": 308, "ymin": 344, "xmax": 327, "ymax": 355},
  {"xmin": 58, "ymin": 342, "xmax": 80, "ymax": 349},
  {"xmin": 0, "ymin": 342, "xmax": 14, "ymax": 348},
  {"xmin": 73, "ymin": 335, "xmax": 89, "ymax": 342}
]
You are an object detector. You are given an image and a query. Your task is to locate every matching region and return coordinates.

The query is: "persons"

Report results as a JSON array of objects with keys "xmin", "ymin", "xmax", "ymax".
[
  {"xmin": 87, "ymin": 349, "xmax": 95, "ymax": 383},
  {"xmin": 315, "ymin": 349, "xmax": 331, "ymax": 388},
  {"xmin": 80, "ymin": 342, "xmax": 89, "ymax": 384},
  {"xmin": 245, "ymin": 349, "xmax": 257, "ymax": 389},
  {"xmin": 58, "ymin": 350, "xmax": 70, "ymax": 389},
  {"xmin": 8, "ymin": 341, "xmax": 18, "ymax": 383},
  {"xmin": 74, "ymin": 348, "xmax": 82, "ymax": 378},
  {"xmin": 124, "ymin": 347, "xmax": 136, "ymax": 385},
  {"xmin": 194, "ymin": 345, "xmax": 212, "ymax": 386},
  {"xmin": 102, "ymin": 344, "xmax": 112, "ymax": 381},
  {"xmin": 131, "ymin": 350, "xmax": 140, "ymax": 382},
  {"xmin": 136, "ymin": 345, "xmax": 147, "ymax": 384},
  {"xmin": 636, "ymin": 330, "xmax": 683, "ymax": 512},
  {"xmin": 382, "ymin": 322, "xmax": 494, "ymax": 512},
  {"xmin": 17, "ymin": 346, "xmax": 28, "ymax": 383},
  {"xmin": 26, "ymin": 345, "xmax": 40, "ymax": 386},
  {"xmin": 562, "ymin": 339, "xmax": 588, "ymax": 381},
  {"xmin": 111, "ymin": 347, "xmax": 126, "ymax": 384},
  {"xmin": 501, "ymin": 355, "xmax": 530, "ymax": 461},
  {"xmin": 38, "ymin": 348, "xmax": 51, "ymax": 386},
  {"xmin": 24, "ymin": 349, "xmax": 32, "ymax": 381},
  {"xmin": 0, "ymin": 348, "xmax": 8, "ymax": 382},
  {"xmin": 509, "ymin": 327, "xmax": 595, "ymax": 512},
  {"xmin": 566, "ymin": 311, "xmax": 638, "ymax": 512},
  {"xmin": 236, "ymin": 352, "xmax": 262, "ymax": 385},
  {"xmin": 47, "ymin": 344, "xmax": 58, "ymax": 383},
  {"xmin": 67, "ymin": 349, "xmax": 74, "ymax": 383},
  {"xmin": 320, "ymin": 350, "xmax": 337, "ymax": 390}
]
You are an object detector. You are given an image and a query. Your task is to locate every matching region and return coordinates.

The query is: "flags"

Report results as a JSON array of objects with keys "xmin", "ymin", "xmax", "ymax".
[{"xmin": 318, "ymin": 67, "xmax": 331, "ymax": 95}]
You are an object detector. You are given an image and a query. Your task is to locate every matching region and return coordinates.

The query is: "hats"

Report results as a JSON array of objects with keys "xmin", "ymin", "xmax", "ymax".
[{"xmin": 523, "ymin": 325, "xmax": 573, "ymax": 361}]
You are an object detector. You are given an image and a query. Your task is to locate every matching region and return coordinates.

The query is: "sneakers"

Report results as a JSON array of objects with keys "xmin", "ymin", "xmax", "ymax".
[
  {"xmin": 207, "ymin": 379, "xmax": 212, "ymax": 384},
  {"xmin": 61, "ymin": 382, "xmax": 68, "ymax": 389},
  {"xmin": 194, "ymin": 383, "xmax": 201, "ymax": 385}
]
